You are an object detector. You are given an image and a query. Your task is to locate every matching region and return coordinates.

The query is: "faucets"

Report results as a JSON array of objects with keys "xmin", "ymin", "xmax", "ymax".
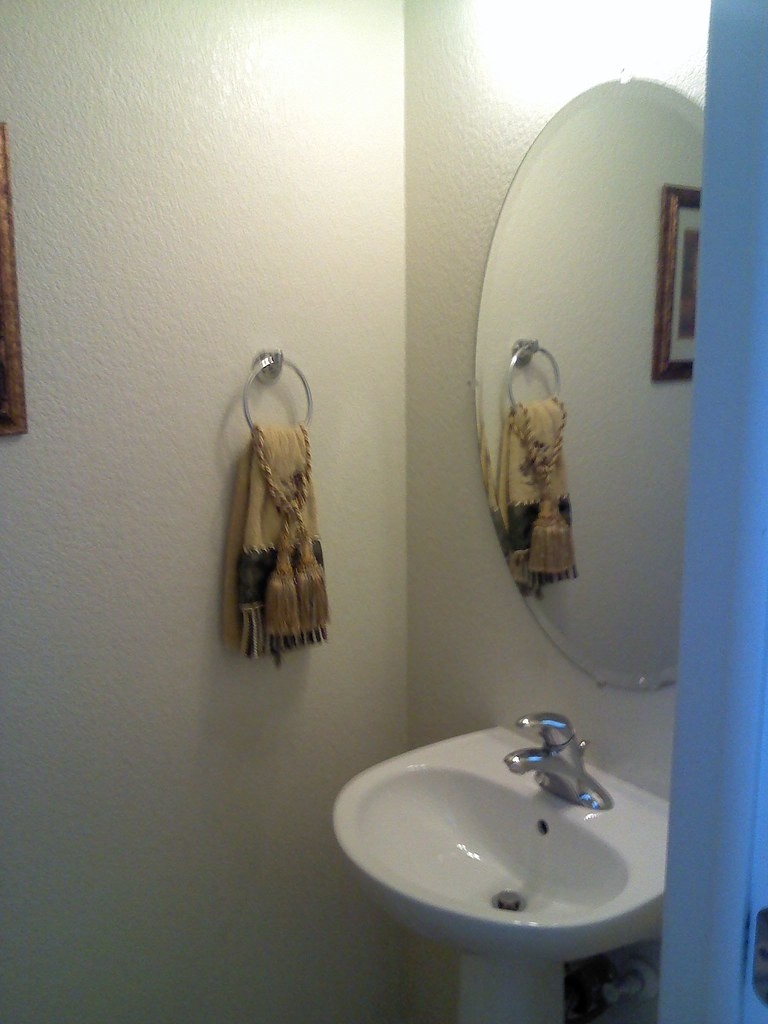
[{"xmin": 502, "ymin": 711, "xmax": 616, "ymax": 811}]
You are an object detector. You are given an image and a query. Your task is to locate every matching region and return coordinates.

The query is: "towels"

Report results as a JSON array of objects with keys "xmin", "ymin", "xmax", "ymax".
[
  {"xmin": 495, "ymin": 395, "xmax": 583, "ymax": 600},
  {"xmin": 221, "ymin": 421, "xmax": 330, "ymax": 669}
]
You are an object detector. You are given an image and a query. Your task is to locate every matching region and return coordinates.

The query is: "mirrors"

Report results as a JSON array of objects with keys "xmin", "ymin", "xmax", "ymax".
[{"xmin": 474, "ymin": 79, "xmax": 703, "ymax": 693}]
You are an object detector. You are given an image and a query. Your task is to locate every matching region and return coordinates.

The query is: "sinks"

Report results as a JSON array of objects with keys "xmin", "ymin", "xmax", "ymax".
[{"xmin": 332, "ymin": 726, "xmax": 672, "ymax": 962}]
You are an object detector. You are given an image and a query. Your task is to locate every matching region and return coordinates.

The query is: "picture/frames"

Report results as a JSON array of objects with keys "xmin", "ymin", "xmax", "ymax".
[{"xmin": 651, "ymin": 184, "xmax": 701, "ymax": 383}]
